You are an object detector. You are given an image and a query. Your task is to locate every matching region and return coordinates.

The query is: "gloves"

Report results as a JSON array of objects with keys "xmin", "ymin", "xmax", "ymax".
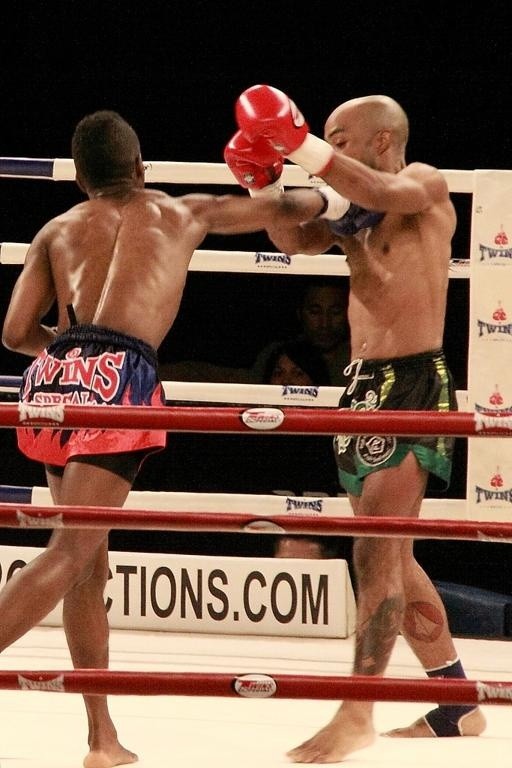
[
  {"xmin": 234, "ymin": 85, "xmax": 334, "ymax": 177},
  {"xmin": 223, "ymin": 129, "xmax": 285, "ymax": 200}
]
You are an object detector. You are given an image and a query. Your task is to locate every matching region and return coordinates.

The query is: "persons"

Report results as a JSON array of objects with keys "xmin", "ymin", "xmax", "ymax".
[
  {"xmin": 1, "ymin": 107, "xmax": 374, "ymax": 763},
  {"xmin": 224, "ymin": 80, "xmax": 488, "ymax": 762},
  {"xmin": 262, "ymin": 344, "xmax": 341, "ymax": 559},
  {"xmin": 299, "ymin": 281, "xmax": 354, "ymax": 385}
]
